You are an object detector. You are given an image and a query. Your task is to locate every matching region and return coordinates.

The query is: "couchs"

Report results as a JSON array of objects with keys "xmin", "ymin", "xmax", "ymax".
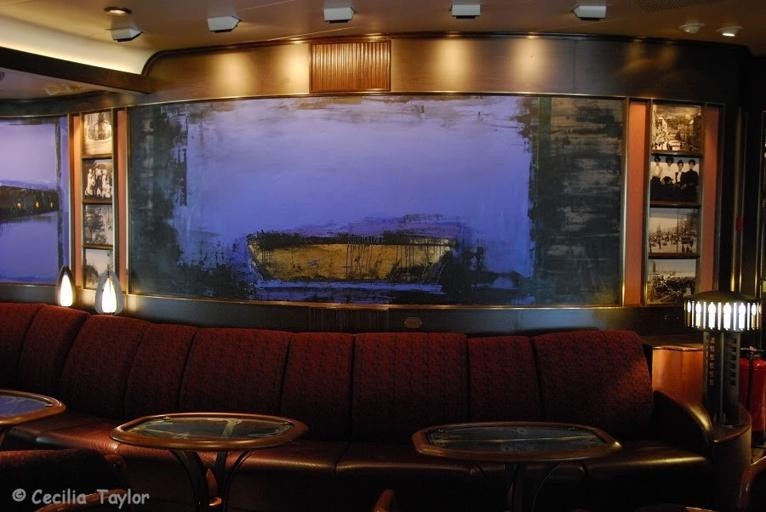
[{"xmin": 0, "ymin": 301, "xmax": 766, "ymax": 512}]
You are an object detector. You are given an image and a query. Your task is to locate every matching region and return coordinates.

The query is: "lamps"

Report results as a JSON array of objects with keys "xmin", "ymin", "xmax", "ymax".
[
  {"xmin": 685, "ymin": 287, "xmax": 762, "ymax": 427},
  {"xmin": 92, "ymin": 269, "xmax": 123, "ymax": 315},
  {"xmin": 55, "ymin": 266, "xmax": 76, "ymax": 307}
]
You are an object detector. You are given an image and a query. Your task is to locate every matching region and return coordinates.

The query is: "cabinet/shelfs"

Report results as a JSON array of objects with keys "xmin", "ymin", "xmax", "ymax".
[{"xmin": 650, "ymin": 346, "xmax": 704, "ymax": 403}]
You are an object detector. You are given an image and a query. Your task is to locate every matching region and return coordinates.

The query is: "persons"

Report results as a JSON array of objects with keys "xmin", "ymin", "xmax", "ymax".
[{"xmin": 650, "ymin": 156, "xmax": 699, "ymax": 200}]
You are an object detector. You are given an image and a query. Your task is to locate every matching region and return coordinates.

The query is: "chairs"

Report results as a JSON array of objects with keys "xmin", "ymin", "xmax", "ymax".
[
  {"xmin": 374, "ymin": 489, "xmax": 397, "ymax": 511},
  {"xmin": 0, "ymin": 448, "xmax": 140, "ymax": 511},
  {"xmin": 684, "ymin": 456, "xmax": 766, "ymax": 512}
]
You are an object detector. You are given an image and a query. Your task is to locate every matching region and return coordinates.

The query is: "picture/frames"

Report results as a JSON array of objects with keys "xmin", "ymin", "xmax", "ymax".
[
  {"xmin": 1, "ymin": 112, "xmax": 71, "ymax": 289},
  {"xmin": 79, "ymin": 107, "xmax": 119, "ymax": 291},
  {"xmin": 127, "ymin": 89, "xmax": 631, "ymax": 313},
  {"xmin": 644, "ymin": 99, "xmax": 707, "ymax": 307}
]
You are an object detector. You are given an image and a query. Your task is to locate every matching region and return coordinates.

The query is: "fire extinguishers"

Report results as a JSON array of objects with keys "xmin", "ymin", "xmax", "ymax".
[{"xmin": 739, "ymin": 347, "xmax": 766, "ymax": 432}]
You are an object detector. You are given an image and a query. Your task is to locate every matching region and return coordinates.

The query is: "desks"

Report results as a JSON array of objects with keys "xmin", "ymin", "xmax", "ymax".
[
  {"xmin": 0, "ymin": 388, "xmax": 66, "ymax": 451},
  {"xmin": 412, "ymin": 420, "xmax": 622, "ymax": 512},
  {"xmin": 705, "ymin": 399, "xmax": 753, "ymax": 498},
  {"xmin": 110, "ymin": 410, "xmax": 308, "ymax": 512}
]
